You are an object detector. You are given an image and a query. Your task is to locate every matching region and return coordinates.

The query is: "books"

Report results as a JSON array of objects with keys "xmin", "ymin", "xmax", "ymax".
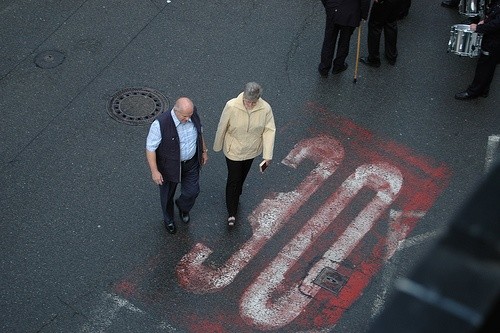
[{"xmin": 260, "ymin": 160, "xmax": 268, "ymax": 172}]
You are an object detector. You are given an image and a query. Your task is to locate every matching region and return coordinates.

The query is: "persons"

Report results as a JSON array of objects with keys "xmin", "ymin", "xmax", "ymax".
[
  {"xmin": 318, "ymin": 0, "xmax": 371, "ymax": 78},
  {"xmin": 455, "ymin": 0, "xmax": 500, "ymax": 101},
  {"xmin": 213, "ymin": 82, "xmax": 276, "ymax": 228},
  {"xmin": 359, "ymin": 0, "xmax": 411, "ymax": 68},
  {"xmin": 146, "ymin": 97, "xmax": 208, "ymax": 234},
  {"xmin": 441, "ymin": 0, "xmax": 481, "ymax": 25}
]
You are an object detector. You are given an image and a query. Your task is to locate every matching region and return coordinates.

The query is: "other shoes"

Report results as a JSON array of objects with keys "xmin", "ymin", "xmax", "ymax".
[
  {"xmin": 317, "ymin": 64, "xmax": 329, "ymax": 78},
  {"xmin": 441, "ymin": 1, "xmax": 459, "ymax": 10},
  {"xmin": 227, "ymin": 215, "xmax": 236, "ymax": 228},
  {"xmin": 332, "ymin": 63, "xmax": 348, "ymax": 75}
]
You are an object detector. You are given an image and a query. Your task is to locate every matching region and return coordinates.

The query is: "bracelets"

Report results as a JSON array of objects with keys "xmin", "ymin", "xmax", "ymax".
[{"xmin": 202, "ymin": 148, "xmax": 208, "ymax": 153}]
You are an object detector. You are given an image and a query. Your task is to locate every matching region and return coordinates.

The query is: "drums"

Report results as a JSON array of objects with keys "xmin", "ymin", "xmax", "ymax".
[
  {"xmin": 448, "ymin": 24, "xmax": 483, "ymax": 58},
  {"xmin": 459, "ymin": 0, "xmax": 486, "ymax": 17}
]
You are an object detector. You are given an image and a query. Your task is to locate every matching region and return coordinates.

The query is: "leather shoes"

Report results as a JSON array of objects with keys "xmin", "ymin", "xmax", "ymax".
[
  {"xmin": 175, "ymin": 198, "xmax": 190, "ymax": 224},
  {"xmin": 385, "ymin": 51, "xmax": 397, "ymax": 65},
  {"xmin": 164, "ymin": 219, "xmax": 177, "ymax": 234},
  {"xmin": 454, "ymin": 91, "xmax": 489, "ymax": 100},
  {"xmin": 359, "ymin": 57, "xmax": 381, "ymax": 67}
]
